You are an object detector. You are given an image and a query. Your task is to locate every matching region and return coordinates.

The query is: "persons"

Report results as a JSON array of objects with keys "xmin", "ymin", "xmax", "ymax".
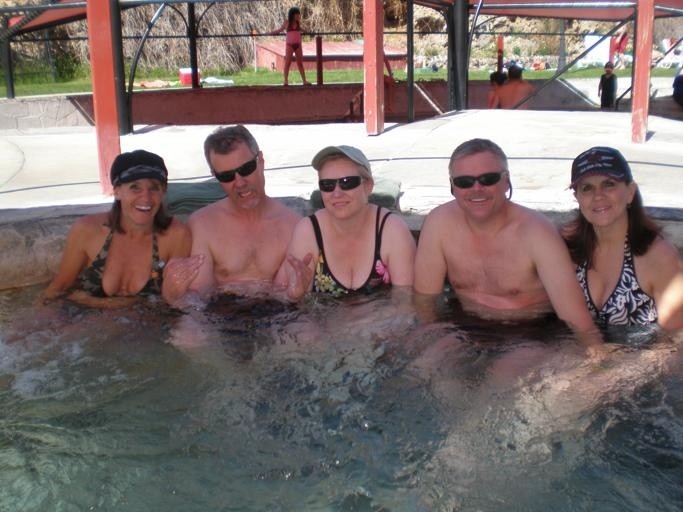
[
  {"xmin": 39, "ymin": 151, "xmax": 192, "ymax": 314},
  {"xmin": 162, "ymin": 124, "xmax": 316, "ymax": 304},
  {"xmin": 276, "ymin": 145, "xmax": 417, "ymax": 304},
  {"xmin": 270, "ymin": 8, "xmax": 313, "ymax": 85},
  {"xmin": 413, "ymin": 139, "xmax": 604, "ymax": 361},
  {"xmin": 672, "ymin": 65, "xmax": 683, "ymax": 105},
  {"xmin": 493, "ymin": 66, "xmax": 536, "ymax": 110},
  {"xmin": 425, "ymin": 56, "xmax": 533, "ymax": 74},
  {"xmin": 561, "ymin": 147, "xmax": 683, "ymax": 349},
  {"xmin": 489, "ymin": 71, "xmax": 507, "ymax": 109},
  {"xmin": 599, "ymin": 62, "xmax": 617, "ymax": 110}
]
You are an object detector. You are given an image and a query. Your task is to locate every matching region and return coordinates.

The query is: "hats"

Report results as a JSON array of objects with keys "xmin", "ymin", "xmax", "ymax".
[
  {"xmin": 568, "ymin": 146, "xmax": 630, "ymax": 187},
  {"xmin": 108, "ymin": 149, "xmax": 171, "ymax": 186},
  {"xmin": 311, "ymin": 145, "xmax": 372, "ymax": 174}
]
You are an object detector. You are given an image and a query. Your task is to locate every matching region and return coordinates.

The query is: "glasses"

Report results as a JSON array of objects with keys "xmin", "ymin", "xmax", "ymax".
[
  {"xmin": 207, "ymin": 155, "xmax": 262, "ymax": 183},
  {"xmin": 317, "ymin": 175, "xmax": 363, "ymax": 193},
  {"xmin": 451, "ymin": 168, "xmax": 507, "ymax": 189}
]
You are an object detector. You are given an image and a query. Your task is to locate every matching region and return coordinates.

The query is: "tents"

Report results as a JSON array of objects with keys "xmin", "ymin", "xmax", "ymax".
[
  {"xmin": 530, "ymin": 62, "xmax": 542, "ymax": 70},
  {"xmin": 540, "ymin": 62, "xmax": 551, "ymax": 70}
]
